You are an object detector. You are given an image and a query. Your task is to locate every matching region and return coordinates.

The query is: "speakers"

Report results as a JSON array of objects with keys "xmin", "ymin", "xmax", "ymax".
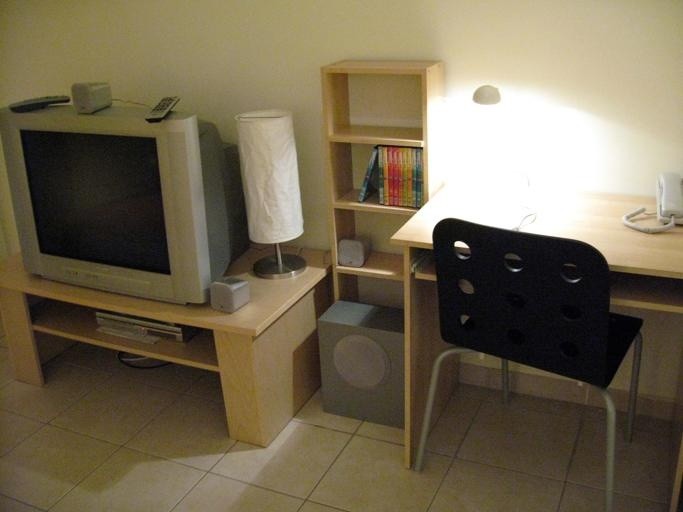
[
  {"xmin": 210, "ymin": 278, "xmax": 251, "ymax": 312},
  {"xmin": 338, "ymin": 233, "xmax": 370, "ymax": 268},
  {"xmin": 317, "ymin": 300, "xmax": 404, "ymax": 429},
  {"xmin": 72, "ymin": 80, "xmax": 110, "ymax": 113}
]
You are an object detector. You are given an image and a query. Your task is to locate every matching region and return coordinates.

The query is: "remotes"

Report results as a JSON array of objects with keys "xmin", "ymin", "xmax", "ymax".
[
  {"xmin": 145, "ymin": 97, "xmax": 179, "ymax": 123},
  {"xmin": 10, "ymin": 95, "xmax": 69, "ymax": 112}
]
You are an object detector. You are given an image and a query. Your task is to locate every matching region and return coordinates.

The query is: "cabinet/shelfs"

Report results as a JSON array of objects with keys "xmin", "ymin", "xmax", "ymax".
[{"xmin": 320, "ymin": 60, "xmax": 446, "ymax": 305}]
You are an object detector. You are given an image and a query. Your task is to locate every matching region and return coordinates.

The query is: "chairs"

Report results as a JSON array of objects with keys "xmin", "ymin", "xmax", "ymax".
[{"xmin": 415, "ymin": 218, "xmax": 645, "ymax": 512}]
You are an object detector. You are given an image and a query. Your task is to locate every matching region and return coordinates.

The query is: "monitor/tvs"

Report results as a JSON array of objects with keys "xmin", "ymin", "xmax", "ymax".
[{"xmin": 0, "ymin": 105, "xmax": 250, "ymax": 306}]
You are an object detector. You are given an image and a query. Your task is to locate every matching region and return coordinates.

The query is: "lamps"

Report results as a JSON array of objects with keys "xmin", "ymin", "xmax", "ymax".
[
  {"xmin": 472, "ymin": 85, "xmax": 500, "ymax": 105},
  {"xmin": 234, "ymin": 109, "xmax": 307, "ymax": 280}
]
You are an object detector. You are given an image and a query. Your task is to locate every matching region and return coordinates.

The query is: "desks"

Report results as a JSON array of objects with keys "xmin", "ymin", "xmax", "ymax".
[
  {"xmin": 387, "ymin": 176, "xmax": 682, "ymax": 512},
  {"xmin": 0, "ymin": 244, "xmax": 333, "ymax": 447}
]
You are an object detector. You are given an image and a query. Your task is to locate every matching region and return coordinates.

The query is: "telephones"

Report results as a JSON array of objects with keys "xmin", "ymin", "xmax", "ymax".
[{"xmin": 656, "ymin": 173, "xmax": 683, "ymax": 225}]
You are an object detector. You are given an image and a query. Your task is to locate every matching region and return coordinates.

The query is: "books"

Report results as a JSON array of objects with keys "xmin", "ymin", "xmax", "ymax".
[{"xmin": 357, "ymin": 145, "xmax": 423, "ymax": 209}]
